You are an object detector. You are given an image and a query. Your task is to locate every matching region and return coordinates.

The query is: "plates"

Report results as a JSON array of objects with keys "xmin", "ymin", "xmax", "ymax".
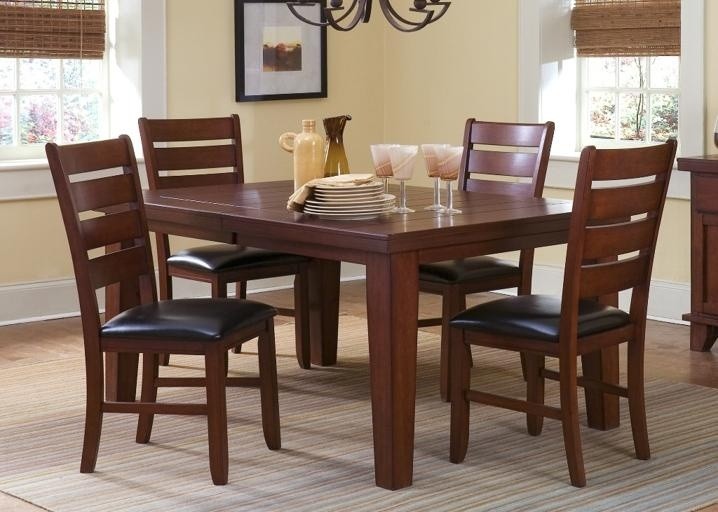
[{"xmin": 301, "ymin": 173, "xmax": 398, "ymax": 221}]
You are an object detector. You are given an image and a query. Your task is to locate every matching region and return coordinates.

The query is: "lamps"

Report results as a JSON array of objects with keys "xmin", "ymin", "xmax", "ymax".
[{"xmin": 288, "ymin": 0, "xmax": 451, "ymax": 33}]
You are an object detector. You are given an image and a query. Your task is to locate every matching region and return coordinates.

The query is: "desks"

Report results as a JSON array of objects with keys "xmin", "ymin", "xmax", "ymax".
[
  {"xmin": 93, "ymin": 177, "xmax": 619, "ymax": 489},
  {"xmin": 672, "ymin": 154, "xmax": 717, "ymax": 351}
]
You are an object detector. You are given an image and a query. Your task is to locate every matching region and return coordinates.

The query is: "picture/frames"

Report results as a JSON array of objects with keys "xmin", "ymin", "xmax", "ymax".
[{"xmin": 234, "ymin": 2, "xmax": 329, "ymax": 101}]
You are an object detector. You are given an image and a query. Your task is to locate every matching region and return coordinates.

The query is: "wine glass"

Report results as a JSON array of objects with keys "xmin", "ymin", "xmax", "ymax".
[
  {"xmin": 366, "ymin": 142, "xmax": 419, "ymax": 216},
  {"xmin": 421, "ymin": 143, "xmax": 465, "ymax": 215}
]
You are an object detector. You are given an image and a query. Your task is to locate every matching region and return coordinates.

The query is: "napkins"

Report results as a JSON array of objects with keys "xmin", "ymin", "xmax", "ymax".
[{"xmin": 286, "ymin": 174, "xmax": 375, "ymax": 214}]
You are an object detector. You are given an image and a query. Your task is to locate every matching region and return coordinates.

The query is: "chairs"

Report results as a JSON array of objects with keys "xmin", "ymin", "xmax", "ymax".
[
  {"xmin": 46, "ymin": 134, "xmax": 281, "ymax": 485},
  {"xmin": 138, "ymin": 112, "xmax": 313, "ymax": 377},
  {"xmin": 419, "ymin": 117, "xmax": 554, "ymax": 403},
  {"xmin": 447, "ymin": 137, "xmax": 678, "ymax": 485}
]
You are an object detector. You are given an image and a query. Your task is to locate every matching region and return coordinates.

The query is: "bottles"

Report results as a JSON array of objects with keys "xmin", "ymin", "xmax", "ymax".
[
  {"xmin": 323, "ymin": 114, "xmax": 352, "ymax": 176},
  {"xmin": 277, "ymin": 120, "xmax": 325, "ymax": 214}
]
